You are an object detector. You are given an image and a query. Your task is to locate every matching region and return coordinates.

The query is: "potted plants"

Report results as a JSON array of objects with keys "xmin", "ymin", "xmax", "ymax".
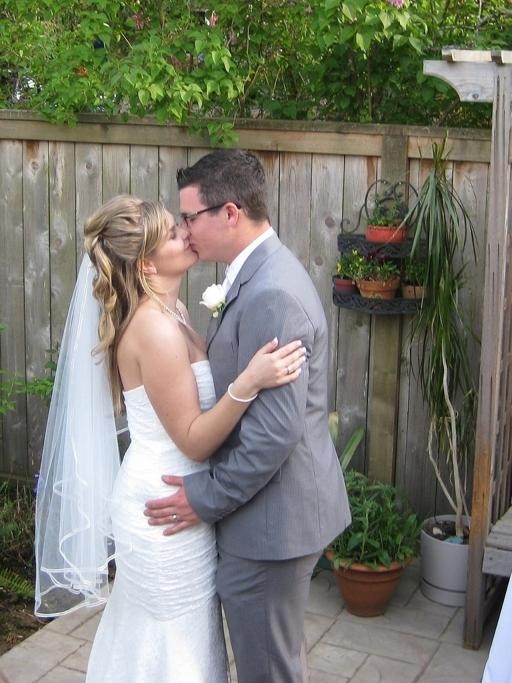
[
  {"xmin": 386, "ymin": 129, "xmax": 497, "ymax": 607},
  {"xmin": 332, "ymin": 248, "xmax": 431, "ymax": 310},
  {"xmin": 363, "ymin": 193, "xmax": 412, "ymax": 254},
  {"xmin": 323, "ymin": 466, "xmax": 427, "ymax": 617}
]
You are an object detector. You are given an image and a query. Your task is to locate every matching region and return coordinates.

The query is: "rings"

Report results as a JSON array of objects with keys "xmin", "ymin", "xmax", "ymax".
[
  {"xmin": 173, "ymin": 515, "xmax": 177, "ymax": 521},
  {"xmin": 287, "ymin": 367, "xmax": 291, "ymax": 376}
]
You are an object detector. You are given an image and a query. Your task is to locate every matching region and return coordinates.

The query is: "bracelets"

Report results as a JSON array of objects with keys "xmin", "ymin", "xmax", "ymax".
[{"xmin": 228, "ymin": 382, "xmax": 258, "ymax": 403}]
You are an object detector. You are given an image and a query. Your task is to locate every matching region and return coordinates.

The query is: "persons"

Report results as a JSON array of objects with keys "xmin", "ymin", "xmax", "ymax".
[
  {"xmin": 33, "ymin": 192, "xmax": 308, "ymax": 683},
  {"xmin": 145, "ymin": 149, "xmax": 354, "ymax": 683}
]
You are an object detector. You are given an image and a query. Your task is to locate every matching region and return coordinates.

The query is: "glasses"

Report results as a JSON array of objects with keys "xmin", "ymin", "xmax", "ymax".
[{"xmin": 181, "ymin": 199, "xmax": 241, "ymax": 227}]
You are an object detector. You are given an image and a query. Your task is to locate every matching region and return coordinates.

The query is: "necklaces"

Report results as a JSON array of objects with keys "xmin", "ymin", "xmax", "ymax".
[{"xmin": 163, "ymin": 305, "xmax": 186, "ymax": 326}]
